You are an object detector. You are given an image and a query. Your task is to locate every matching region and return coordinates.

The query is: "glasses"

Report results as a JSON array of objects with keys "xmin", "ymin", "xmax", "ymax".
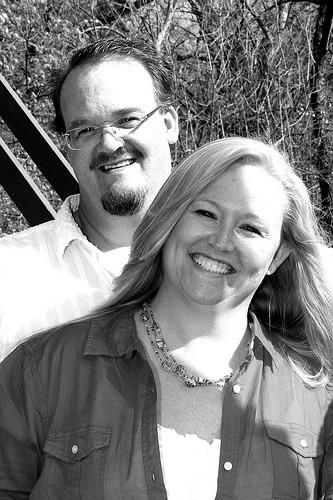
[{"xmin": 60, "ymin": 106, "xmax": 160, "ymax": 151}]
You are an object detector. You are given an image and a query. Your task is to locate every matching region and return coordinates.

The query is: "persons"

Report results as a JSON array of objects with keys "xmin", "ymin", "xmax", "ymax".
[
  {"xmin": 0, "ymin": 42, "xmax": 333, "ymax": 362},
  {"xmin": 0, "ymin": 138, "xmax": 333, "ymax": 500}
]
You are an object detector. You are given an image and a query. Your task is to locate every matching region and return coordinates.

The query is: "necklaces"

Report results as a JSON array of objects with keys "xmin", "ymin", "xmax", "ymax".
[{"xmin": 138, "ymin": 299, "xmax": 229, "ymax": 388}]
